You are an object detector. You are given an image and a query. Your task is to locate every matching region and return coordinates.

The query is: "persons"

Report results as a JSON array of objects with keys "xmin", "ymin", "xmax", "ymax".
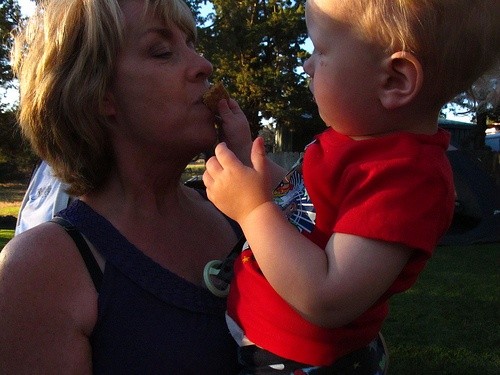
[
  {"xmin": 0, "ymin": 0, "xmax": 238, "ymax": 375},
  {"xmin": 202, "ymin": 0, "xmax": 500, "ymax": 375}
]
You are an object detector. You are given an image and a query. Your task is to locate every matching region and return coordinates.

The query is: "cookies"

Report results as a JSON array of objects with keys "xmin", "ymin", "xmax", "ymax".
[{"xmin": 202, "ymin": 82, "xmax": 230, "ymax": 112}]
